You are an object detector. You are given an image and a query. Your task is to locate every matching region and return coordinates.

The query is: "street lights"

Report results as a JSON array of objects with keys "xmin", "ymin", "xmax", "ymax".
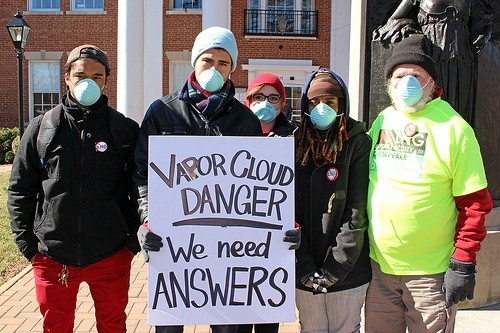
[{"xmin": 5, "ymin": 9, "xmax": 32, "ymax": 141}]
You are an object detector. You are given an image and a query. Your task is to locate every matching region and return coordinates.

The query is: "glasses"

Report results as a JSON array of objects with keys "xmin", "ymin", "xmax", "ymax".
[{"xmin": 252, "ymin": 93, "xmax": 282, "ymax": 104}]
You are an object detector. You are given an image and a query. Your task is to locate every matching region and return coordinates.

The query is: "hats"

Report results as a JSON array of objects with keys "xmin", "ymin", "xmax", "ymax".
[
  {"xmin": 191, "ymin": 27, "xmax": 238, "ymax": 74},
  {"xmin": 246, "ymin": 73, "xmax": 286, "ymax": 111},
  {"xmin": 306, "ymin": 74, "xmax": 344, "ymax": 103},
  {"xmin": 384, "ymin": 34, "xmax": 438, "ymax": 82},
  {"xmin": 67, "ymin": 45, "xmax": 110, "ymax": 69}
]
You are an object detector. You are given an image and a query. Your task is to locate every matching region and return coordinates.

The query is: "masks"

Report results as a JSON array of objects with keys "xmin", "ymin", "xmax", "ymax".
[
  {"xmin": 198, "ymin": 68, "xmax": 226, "ymax": 93},
  {"xmin": 67, "ymin": 79, "xmax": 105, "ymax": 107},
  {"xmin": 388, "ymin": 75, "xmax": 430, "ymax": 107},
  {"xmin": 251, "ymin": 102, "xmax": 277, "ymax": 123},
  {"xmin": 304, "ymin": 103, "xmax": 345, "ymax": 131}
]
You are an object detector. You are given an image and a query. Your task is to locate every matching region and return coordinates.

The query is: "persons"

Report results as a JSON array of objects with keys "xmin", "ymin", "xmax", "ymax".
[
  {"xmin": 7, "ymin": 44, "xmax": 139, "ymax": 333},
  {"xmin": 133, "ymin": 26, "xmax": 301, "ymax": 333},
  {"xmin": 365, "ymin": 34, "xmax": 493, "ymax": 333},
  {"xmin": 372, "ymin": 0, "xmax": 497, "ymax": 131},
  {"xmin": 290, "ymin": 68, "xmax": 373, "ymax": 333},
  {"xmin": 245, "ymin": 73, "xmax": 301, "ymax": 333}
]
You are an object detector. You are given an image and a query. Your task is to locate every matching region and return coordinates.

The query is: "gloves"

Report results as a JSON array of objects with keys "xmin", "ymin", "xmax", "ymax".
[
  {"xmin": 442, "ymin": 258, "xmax": 477, "ymax": 304},
  {"xmin": 311, "ymin": 267, "xmax": 339, "ymax": 292},
  {"xmin": 282, "ymin": 222, "xmax": 302, "ymax": 250},
  {"xmin": 136, "ymin": 224, "xmax": 163, "ymax": 263},
  {"xmin": 298, "ymin": 270, "xmax": 327, "ymax": 293}
]
